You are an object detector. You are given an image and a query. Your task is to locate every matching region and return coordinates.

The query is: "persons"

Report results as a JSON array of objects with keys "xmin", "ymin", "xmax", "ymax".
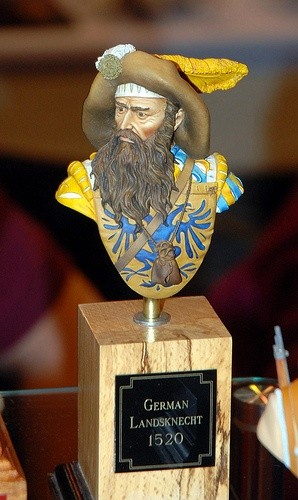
[
  {"xmin": 0, "ymin": 173, "xmax": 111, "ymax": 500},
  {"xmin": 54, "ymin": 43, "xmax": 250, "ymax": 301}
]
